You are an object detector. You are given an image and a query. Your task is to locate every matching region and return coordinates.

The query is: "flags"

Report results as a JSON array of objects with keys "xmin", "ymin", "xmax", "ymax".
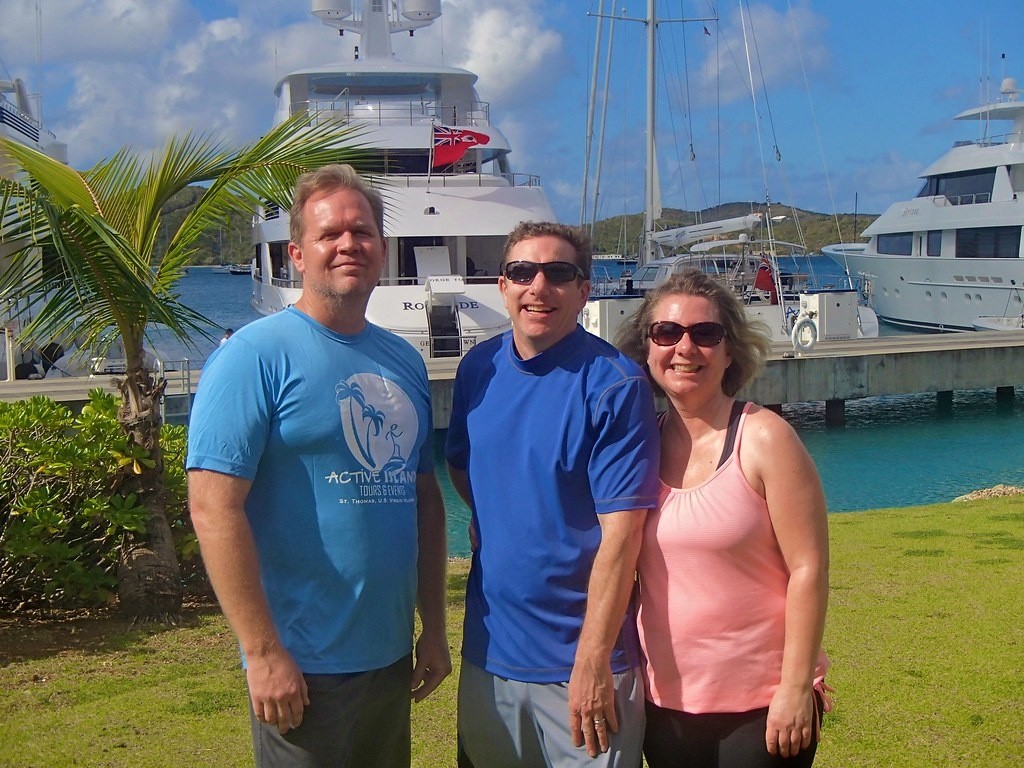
[
  {"xmin": 431, "ymin": 124, "xmax": 490, "ymax": 168},
  {"xmin": 755, "ymin": 258, "xmax": 779, "ymax": 304}
]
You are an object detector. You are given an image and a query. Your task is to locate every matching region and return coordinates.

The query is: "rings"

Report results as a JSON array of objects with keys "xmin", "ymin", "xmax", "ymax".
[{"xmin": 594, "ymin": 719, "xmax": 605, "ymax": 725}]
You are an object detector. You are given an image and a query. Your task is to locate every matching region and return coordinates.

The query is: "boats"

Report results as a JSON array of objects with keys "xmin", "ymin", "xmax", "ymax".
[
  {"xmin": 819, "ymin": 76, "xmax": 1024, "ymax": 335},
  {"xmin": 212, "ymin": 263, "xmax": 251, "ymax": 274},
  {"xmin": 248, "ymin": 0, "xmax": 566, "ymax": 377}
]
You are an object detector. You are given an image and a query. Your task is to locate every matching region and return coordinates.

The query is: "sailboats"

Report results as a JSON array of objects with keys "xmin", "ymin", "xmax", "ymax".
[{"xmin": 579, "ymin": 0, "xmax": 879, "ymax": 345}]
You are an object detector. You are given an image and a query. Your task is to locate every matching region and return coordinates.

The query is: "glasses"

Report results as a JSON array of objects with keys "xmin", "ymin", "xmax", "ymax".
[
  {"xmin": 503, "ymin": 260, "xmax": 586, "ymax": 284},
  {"xmin": 646, "ymin": 320, "xmax": 729, "ymax": 347}
]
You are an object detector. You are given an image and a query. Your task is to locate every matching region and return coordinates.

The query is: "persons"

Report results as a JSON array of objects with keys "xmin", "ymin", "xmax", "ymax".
[
  {"xmin": 219, "ymin": 327, "xmax": 234, "ymax": 347},
  {"xmin": 185, "ymin": 163, "xmax": 456, "ymax": 768},
  {"xmin": 450, "ymin": 219, "xmax": 834, "ymax": 768}
]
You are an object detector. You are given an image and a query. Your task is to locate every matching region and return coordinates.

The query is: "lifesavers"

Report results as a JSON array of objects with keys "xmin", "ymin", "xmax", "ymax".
[{"xmin": 790, "ymin": 316, "xmax": 818, "ymax": 355}]
